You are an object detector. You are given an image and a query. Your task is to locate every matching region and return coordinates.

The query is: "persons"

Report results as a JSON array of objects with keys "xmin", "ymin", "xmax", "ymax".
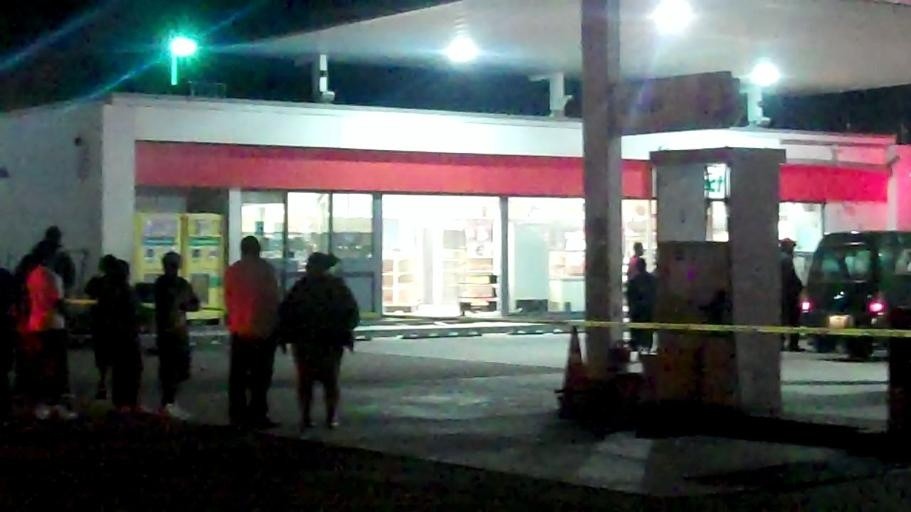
[
  {"xmin": 224, "ymin": 236, "xmax": 285, "ymax": 429},
  {"xmin": 626, "ymin": 243, "xmax": 656, "ymax": 350},
  {"xmin": 780, "ymin": 238, "xmax": 806, "ymax": 353},
  {"xmin": 0, "ymin": 226, "xmax": 200, "ymax": 421},
  {"xmin": 277, "ymin": 253, "xmax": 361, "ymax": 429}
]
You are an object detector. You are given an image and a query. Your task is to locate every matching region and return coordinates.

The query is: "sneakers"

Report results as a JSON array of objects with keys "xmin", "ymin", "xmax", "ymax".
[
  {"xmin": 33, "ymin": 393, "xmax": 79, "ymax": 420},
  {"xmin": 780, "ymin": 343, "xmax": 806, "ymax": 352},
  {"xmin": 301, "ymin": 407, "xmax": 341, "ymax": 427},
  {"xmin": 94, "ymin": 379, "xmax": 111, "ymax": 400},
  {"xmin": 229, "ymin": 409, "xmax": 281, "ymax": 431},
  {"xmin": 113, "ymin": 401, "xmax": 192, "ymax": 423}
]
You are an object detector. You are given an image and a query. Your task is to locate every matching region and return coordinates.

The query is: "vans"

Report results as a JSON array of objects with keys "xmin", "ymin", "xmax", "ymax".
[{"xmin": 800, "ymin": 231, "xmax": 911, "ymax": 351}]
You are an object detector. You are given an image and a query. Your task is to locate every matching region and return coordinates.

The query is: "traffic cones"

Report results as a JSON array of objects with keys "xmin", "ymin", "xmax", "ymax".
[{"xmin": 553, "ymin": 325, "xmax": 590, "ymax": 393}]
